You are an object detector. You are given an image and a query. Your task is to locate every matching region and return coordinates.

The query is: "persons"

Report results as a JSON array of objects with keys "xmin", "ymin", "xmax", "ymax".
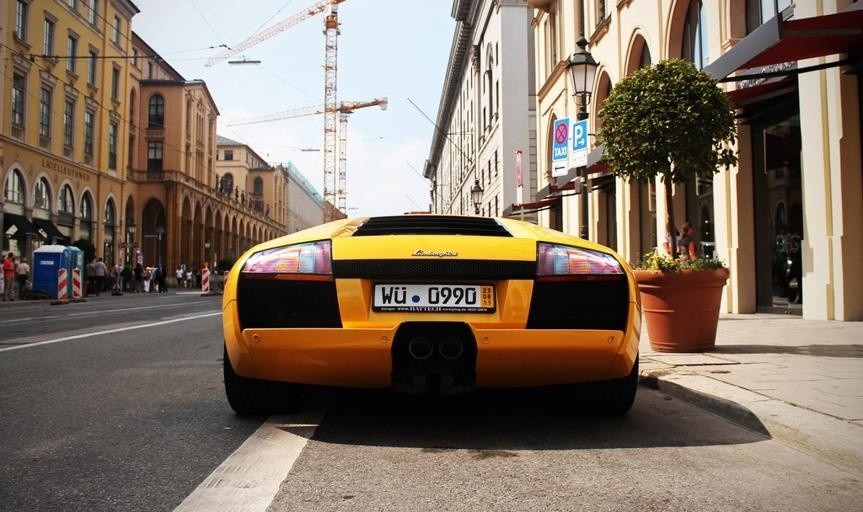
[
  {"xmin": 14, "ymin": 256, "xmax": 30, "ymax": 298},
  {"xmin": 662, "ymin": 221, "xmax": 681, "ymax": 257},
  {"xmin": 1, "ymin": 252, "xmax": 14, "ymax": 302},
  {"xmin": 675, "ymin": 222, "xmax": 699, "ymax": 261},
  {"xmin": 0, "ymin": 255, "xmax": 7, "ymax": 296},
  {"xmin": 84, "ymin": 256, "xmax": 200, "ymax": 296}
]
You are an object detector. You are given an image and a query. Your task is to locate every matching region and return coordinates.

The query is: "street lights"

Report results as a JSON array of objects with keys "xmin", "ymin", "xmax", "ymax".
[
  {"xmin": 156, "ymin": 224, "xmax": 166, "ymax": 266},
  {"xmin": 470, "ymin": 176, "xmax": 485, "ymax": 213},
  {"xmin": 127, "ymin": 222, "xmax": 137, "ymax": 273},
  {"xmin": 560, "ymin": 33, "xmax": 602, "ymax": 246},
  {"xmin": 202, "ymin": 238, "xmax": 211, "ymax": 262}
]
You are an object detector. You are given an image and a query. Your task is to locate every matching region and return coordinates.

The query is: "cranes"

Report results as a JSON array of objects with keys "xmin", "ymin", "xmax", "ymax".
[
  {"xmin": 223, "ymin": 96, "xmax": 389, "ymax": 220},
  {"xmin": 197, "ymin": 0, "xmax": 346, "ymax": 222}
]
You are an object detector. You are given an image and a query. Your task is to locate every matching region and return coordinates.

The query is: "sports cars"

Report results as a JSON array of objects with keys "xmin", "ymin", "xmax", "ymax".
[{"xmin": 216, "ymin": 214, "xmax": 642, "ymax": 421}]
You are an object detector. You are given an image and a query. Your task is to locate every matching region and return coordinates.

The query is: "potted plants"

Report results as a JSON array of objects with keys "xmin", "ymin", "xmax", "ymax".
[{"xmin": 588, "ymin": 58, "xmax": 740, "ymax": 354}]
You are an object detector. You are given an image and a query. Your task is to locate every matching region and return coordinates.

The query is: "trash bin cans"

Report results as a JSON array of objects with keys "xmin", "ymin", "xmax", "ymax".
[{"xmin": 112, "ymin": 277, "xmax": 123, "ymax": 296}]
